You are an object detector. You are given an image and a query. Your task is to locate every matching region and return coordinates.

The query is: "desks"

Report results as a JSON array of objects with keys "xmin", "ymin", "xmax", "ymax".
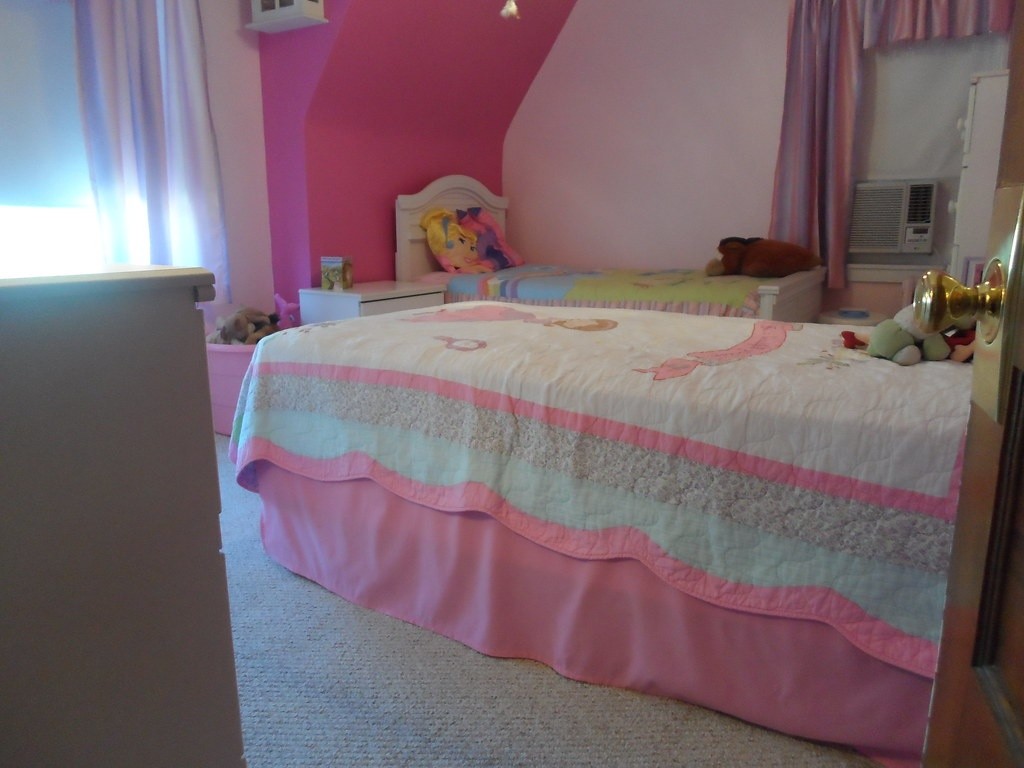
[
  {"xmin": 818, "ymin": 307, "xmax": 887, "ymax": 327},
  {"xmin": 846, "ymin": 264, "xmax": 935, "ymax": 308}
]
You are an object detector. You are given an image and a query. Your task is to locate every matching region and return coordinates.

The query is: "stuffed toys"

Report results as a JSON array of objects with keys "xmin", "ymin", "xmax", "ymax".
[
  {"xmin": 203, "ymin": 307, "xmax": 280, "ymax": 346},
  {"xmin": 841, "ymin": 301, "xmax": 976, "ymax": 367},
  {"xmin": 704, "ymin": 236, "xmax": 822, "ymax": 278}
]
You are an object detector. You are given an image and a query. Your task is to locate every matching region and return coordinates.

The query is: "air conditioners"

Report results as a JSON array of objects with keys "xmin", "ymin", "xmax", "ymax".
[{"xmin": 845, "ymin": 180, "xmax": 939, "ymax": 254}]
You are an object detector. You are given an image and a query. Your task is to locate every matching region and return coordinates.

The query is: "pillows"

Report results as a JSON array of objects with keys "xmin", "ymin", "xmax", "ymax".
[{"xmin": 418, "ymin": 206, "xmax": 526, "ymax": 276}]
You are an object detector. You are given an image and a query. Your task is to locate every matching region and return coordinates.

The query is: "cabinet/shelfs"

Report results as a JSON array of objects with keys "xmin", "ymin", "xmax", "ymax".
[
  {"xmin": 0, "ymin": 268, "xmax": 251, "ymax": 768},
  {"xmin": 947, "ymin": 69, "xmax": 1011, "ymax": 288}
]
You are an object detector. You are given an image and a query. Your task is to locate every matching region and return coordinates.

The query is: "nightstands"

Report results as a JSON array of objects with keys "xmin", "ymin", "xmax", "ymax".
[{"xmin": 297, "ymin": 279, "xmax": 447, "ymax": 325}]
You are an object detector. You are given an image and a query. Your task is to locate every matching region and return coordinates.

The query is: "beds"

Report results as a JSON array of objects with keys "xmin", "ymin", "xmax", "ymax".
[
  {"xmin": 229, "ymin": 299, "xmax": 1005, "ymax": 767},
  {"xmin": 393, "ymin": 174, "xmax": 827, "ymax": 324}
]
